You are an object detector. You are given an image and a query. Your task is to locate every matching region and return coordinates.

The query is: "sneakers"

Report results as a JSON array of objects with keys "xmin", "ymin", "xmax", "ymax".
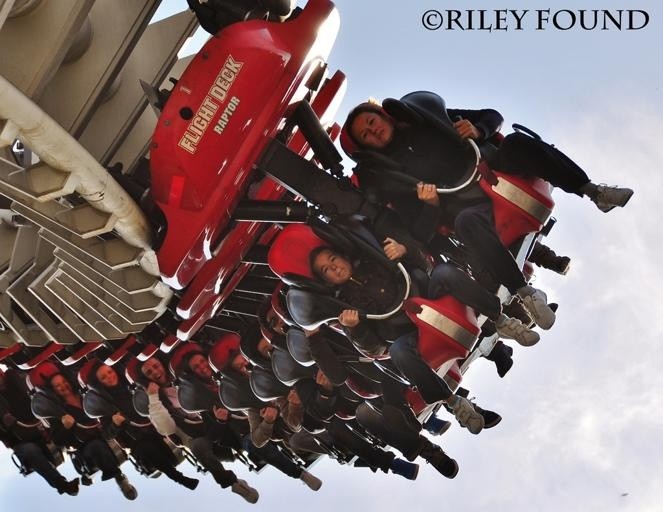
[
  {"xmin": 232, "ymin": 480, "xmax": 259, "ymax": 503},
  {"xmin": 453, "ymin": 397, "xmax": 502, "ymax": 434},
  {"xmin": 495, "ymin": 345, "xmax": 514, "ymax": 377},
  {"xmin": 400, "ymin": 460, "xmax": 419, "ymax": 479},
  {"xmin": 553, "ymin": 257, "xmax": 571, "ymax": 274},
  {"xmin": 495, "ymin": 288, "xmax": 558, "ymax": 347},
  {"xmin": 118, "ymin": 473, "xmax": 137, "ymax": 500},
  {"xmin": 593, "ymin": 185, "xmax": 634, "ymax": 212},
  {"xmin": 301, "ymin": 471, "xmax": 322, "ymax": 491},
  {"xmin": 180, "ymin": 477, "xmax": 199, "ymax": 490},
  {"xmin": 420, "ymin": 441, "xmax": 459, "ymax": 479},
  {"xmin": 425, "ymin": 417, "xmax": 451, "ymax": 435},
  {"xmin": 58, "ymin": 477, "xmax": 79, "ymax": 496}
]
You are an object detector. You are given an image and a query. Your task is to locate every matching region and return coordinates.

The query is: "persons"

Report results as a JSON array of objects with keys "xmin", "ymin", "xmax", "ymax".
[
  {"xmin": 139, "ymin": 356, "xmax": 258, "ymax": 503},
  {"xmin": 255, "ymin": 336, "xmax": 452, "ymax": 481},
  {"xmin": 88, "ymin": 361, "xmax": 199, "ymax": 488},
  {"xmin": 479, "ymin": 242, "xmax": 570, "ymax": 378},
  {"xmin": 226, "ymin": 350, "xmax": 325, "ymax": 456},
  {"xmin": 182, "ymin": 350, "xmax": 322, "ymax": 492},
  {"xmin": 1, "ymin": 370, "xmax": 78, "ymax": 494},
  {"xmin": 309, "ymin": 238, "xmax": 541, "ymax": 433},
  {"xmin": 260, "ymin": 303, "xmax": 502, "ymax": 478},
  {"xmin": 345, "ymin": 102, "xmax": 634, "ymax": 329},
  {"xmin": 49, "ymin": 372, "xmax": 138, "ymax": 501}
]
